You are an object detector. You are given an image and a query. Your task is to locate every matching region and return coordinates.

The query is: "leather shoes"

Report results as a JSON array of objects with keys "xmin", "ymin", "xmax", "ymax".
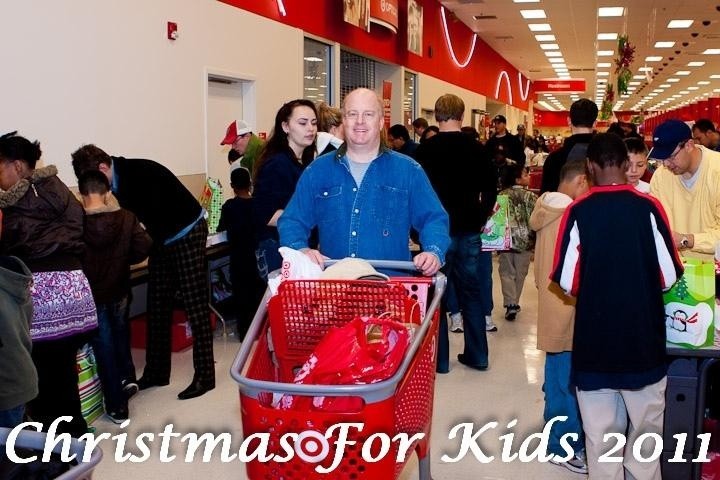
[
  {"xmin": 177, "ymin": 374, "xmax": 215, "ymax": 399},
  {"xmin": 137, "ymin": 376, "xmax": 169, "ymax": 390}
]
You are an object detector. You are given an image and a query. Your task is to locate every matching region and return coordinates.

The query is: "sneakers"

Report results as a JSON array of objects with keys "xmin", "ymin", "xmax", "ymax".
[
  {"xmin": 546, "ymin": 447, "xmax": 588, "ymax": 474},
  {"xmin": 505, "ymin": 304, "xmax": 521, "ymax": 320},
  {"xmin": 448, "ymin": 311, "xmax": 464, "ymax": 333},
  {"xmin": 122, "ymin": 379, "xmax": 139, "ymax": 400},
  {"xmin": 106, "ymin": 413, "xmax": 128, "ymax": 424},
  {"xmin": 458, "ymin": 354, "xmax": 488, "ymax": 368},
  {"xmin": 485, "ymin": 315, "xmax": 497, "ymax": 331}
]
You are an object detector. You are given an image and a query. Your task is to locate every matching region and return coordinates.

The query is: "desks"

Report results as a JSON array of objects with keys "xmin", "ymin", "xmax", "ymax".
[
  {"xmin": 130, "ymin": 230, "xmax": 230, "ymax": 280},
  {"xmin": 660, "ymin": 347, "xmax": 720, "ymax": 480}
]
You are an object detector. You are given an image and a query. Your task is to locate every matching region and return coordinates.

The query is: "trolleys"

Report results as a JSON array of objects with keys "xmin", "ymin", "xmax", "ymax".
[{"xmin": 227, "ymin": 256, "xmax": 447, "ymax": 479}]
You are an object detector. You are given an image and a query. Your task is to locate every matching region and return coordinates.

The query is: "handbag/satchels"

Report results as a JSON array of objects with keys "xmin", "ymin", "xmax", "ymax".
[
  {"xmin": 28, "ymin": 269, "xmax": 98, "ymax": 342},
  {"xmin": 662, "ymin": 256, "xmax": 716, "ymax": 348},
  {"xmin": 75, "ymin": 346, "xmax": 105, "ymax": 426},
  {"xmin": 480, "ymin": 195, "xmax": 513, "ymax": 252}
]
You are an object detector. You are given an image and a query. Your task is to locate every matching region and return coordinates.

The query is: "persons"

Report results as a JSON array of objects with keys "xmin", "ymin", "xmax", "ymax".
[
  {"xmin": 0, "ymin": 255, "xmax": 40, "ymax": 428},
  {"xmin": 72, "ymin": 144, "xmax": 216, "ymax": 399},
  {"xmin": 77, "ymin": 168, "xmax": 154, "ymax": 422},
  {"xmin": 218, "ymin": 87, "xmax": 719, "ymax": 480},
  {"xmin": 1, "ymin": 130, "xmax": 98, "ymax": 440}
]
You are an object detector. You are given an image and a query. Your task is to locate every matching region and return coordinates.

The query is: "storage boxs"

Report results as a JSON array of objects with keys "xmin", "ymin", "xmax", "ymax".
[{"xmin": 129, "ymin": 310, "xmax": 216, "ymax": 352}]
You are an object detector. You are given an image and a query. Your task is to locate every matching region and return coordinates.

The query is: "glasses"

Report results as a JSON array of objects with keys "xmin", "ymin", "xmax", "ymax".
[{"xmin": 668, "ymin": 143, "xmax": 685, "ymax": 159}]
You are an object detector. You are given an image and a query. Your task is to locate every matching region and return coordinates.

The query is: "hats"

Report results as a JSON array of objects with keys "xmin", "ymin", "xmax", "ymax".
[
  {"xmin": 517, "ymin": 124, "xmax": 526, "ymax": 130},
  {"xmin": 220, "ymin": 120, "xmax": 252, "ymax": 145},
  {"xmin": 492, "ymin": 115, "xmax": 507, "ymax": 123},
  {"xmin": 646, "ymin": 119, "xmax": 691, "ymax": 160},
  {"xmin": 230, "ymin": 167, "xmax": 252, "ymax": 184}
]
